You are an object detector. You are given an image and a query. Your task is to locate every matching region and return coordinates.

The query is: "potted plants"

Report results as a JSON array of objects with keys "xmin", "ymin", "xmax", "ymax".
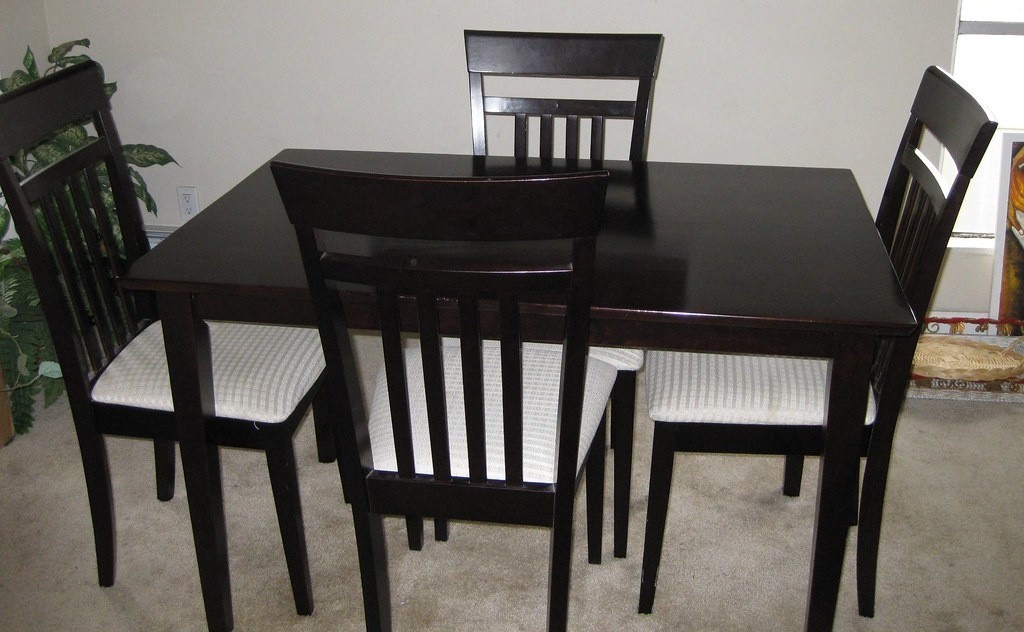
[{"xmin": 0, "ymin": 37, "xmax": 181, "ymax": 449}]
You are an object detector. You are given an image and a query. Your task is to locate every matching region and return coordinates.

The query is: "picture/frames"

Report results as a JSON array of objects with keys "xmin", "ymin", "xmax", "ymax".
[{"xmin": 988, "ymin": 133, "xmax": 1024, "ymax": 336}]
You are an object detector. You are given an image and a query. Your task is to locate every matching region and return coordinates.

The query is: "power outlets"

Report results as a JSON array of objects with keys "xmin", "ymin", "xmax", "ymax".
[{"xmin": 177, "ymin": 186, "xmax": 198, "ymax": 220}]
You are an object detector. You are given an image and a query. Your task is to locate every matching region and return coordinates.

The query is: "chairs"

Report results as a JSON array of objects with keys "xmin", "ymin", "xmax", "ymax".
[{"xmin": 0, "ymin": 30, "xmax": 999, "ymax": 632}]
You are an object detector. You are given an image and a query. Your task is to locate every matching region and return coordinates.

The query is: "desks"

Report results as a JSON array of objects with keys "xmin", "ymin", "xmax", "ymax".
[{"xmin": 124, "ymin": 149, "xmax": 917, "ymax": 632}]
